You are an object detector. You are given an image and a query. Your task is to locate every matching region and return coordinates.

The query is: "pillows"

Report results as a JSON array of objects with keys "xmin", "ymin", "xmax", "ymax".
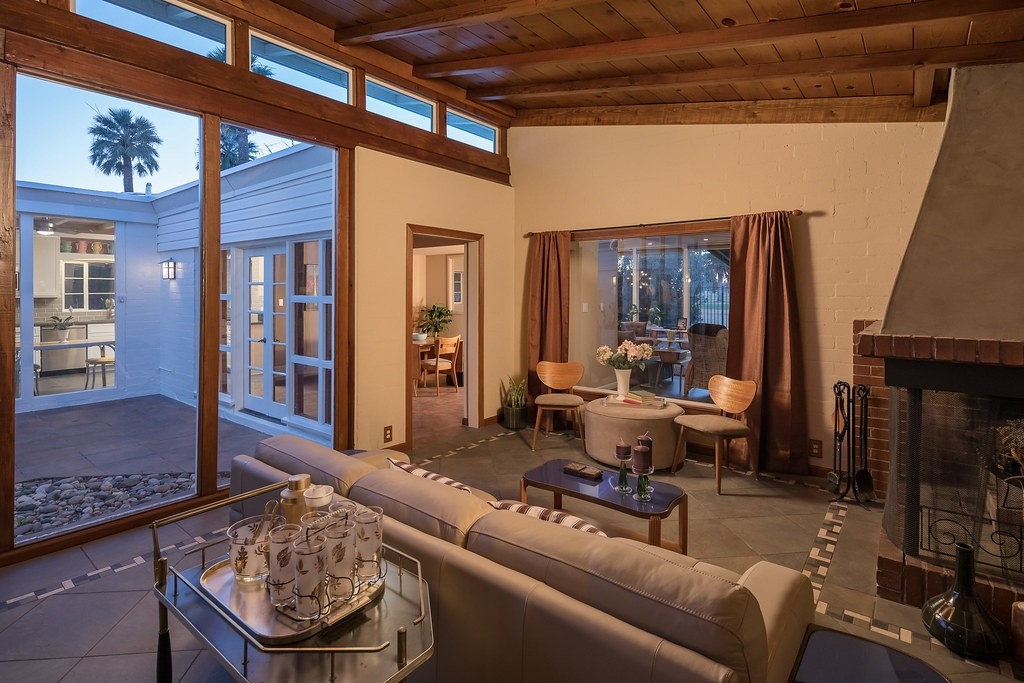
[
  {"xmin": 488, "ymin": 497, "xmax": 606, "ymax": 535},
  {"xmin": 386, "ymin": 456, "xmax": 468, "ymax": 490}
]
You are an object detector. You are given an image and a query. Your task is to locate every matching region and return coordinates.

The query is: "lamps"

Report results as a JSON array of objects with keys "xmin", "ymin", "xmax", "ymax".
[
  {"xmin": 37, "ymin": 217, "xmax": 56, "ymax": 235},
  {"xmin": 158, "ymin": 257, "xmax": 175, "ymax": 282}
]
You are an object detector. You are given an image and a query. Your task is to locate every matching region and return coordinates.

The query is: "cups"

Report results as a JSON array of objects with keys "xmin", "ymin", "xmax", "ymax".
[{"xmin": 269, "ymin": 484, "xmax": 384, "ymax": 621}]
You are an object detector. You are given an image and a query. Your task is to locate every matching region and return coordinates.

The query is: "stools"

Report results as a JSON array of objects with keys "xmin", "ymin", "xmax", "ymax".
[
  {"xmin": 85, "ymin": 356, "xmax": 115, "ymax": 388},
  {"xmin": 16, "ymin": 362, "xmax": 41, "ymax": 395}
]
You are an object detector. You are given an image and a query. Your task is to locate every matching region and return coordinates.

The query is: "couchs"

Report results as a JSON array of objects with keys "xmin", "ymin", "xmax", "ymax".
[{"xmin": 232, "ymin": 436, "xmax": 816, "ymax": 683}]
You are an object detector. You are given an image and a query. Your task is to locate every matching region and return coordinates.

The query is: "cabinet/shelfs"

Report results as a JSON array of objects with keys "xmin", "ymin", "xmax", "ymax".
[{"xmin": 15, "ymin": 235, "xmax": 62, "ymax": 299}]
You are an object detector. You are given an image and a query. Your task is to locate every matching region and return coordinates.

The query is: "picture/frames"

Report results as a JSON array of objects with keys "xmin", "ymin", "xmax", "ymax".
[
  {"xmin": 305, "ymin": 264, "xmax": 321, "ymax": 310},
  {"xmin": 677, "ymin": 317, "xmax": 687, "ymax": 329}
]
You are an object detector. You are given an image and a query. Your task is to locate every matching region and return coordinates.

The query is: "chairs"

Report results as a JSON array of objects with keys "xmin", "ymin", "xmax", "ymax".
[
  {"xmin": 617, "ymin": 322, "xmax": 674, "ymax": 390},
  {"xmin": 532, "ymin": 360, "xmax": 585, "ymax": 452},
  {"xmin": 421, "ymin": 334, "xmax": 462, "ymax": 396},
  {"xmin": 674, "ymin": 374, "xmax": 758, "ymax": 493},
  {"xmin": 683, "ymin": 323, "xmax": 729, "ymax": 395}
]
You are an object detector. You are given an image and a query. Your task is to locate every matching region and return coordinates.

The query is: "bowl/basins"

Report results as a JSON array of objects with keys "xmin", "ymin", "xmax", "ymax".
[
  {"xmin": 226, "ymin": 514, "xmax": 286, "ymax": 583},
  {"xmin": 413, "ymin": 333, "xmax": 427, "ymax": 340}
]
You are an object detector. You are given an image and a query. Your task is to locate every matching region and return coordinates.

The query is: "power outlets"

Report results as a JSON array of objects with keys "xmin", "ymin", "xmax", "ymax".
[
  {"xmin": 808, "ymin": 439, "xmax": 821, "ymax": 459},
  {"xmin": 383, "ymin": 425, "xmax": 394, "ymax": 445}
]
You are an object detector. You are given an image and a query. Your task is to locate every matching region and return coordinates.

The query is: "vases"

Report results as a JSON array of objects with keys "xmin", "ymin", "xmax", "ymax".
[
  {"xmin": 615, "ymin": 367, "xmax": 632, "ymax": 395},
  {"xmin": 920, "ymin": 543, "xmax": 1011, "ymax": 662}
]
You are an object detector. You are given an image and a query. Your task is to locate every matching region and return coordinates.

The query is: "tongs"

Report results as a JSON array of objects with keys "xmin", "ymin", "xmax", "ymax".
[{"xmin": 238, "ymin": 498, "xmax": 279, "ymax": 572}]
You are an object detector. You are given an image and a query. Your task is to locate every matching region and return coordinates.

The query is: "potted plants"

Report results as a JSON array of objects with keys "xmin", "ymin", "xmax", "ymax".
[
  {"xmin": 500, "ymin": 373, "xmax": 529, "ymax": 429},
  {"xmin": 51, "ymin": 314, "xmax": 76, "ymax": 341},
  {"xmin": 420, "ymin": 304, "xmax": 451, "ymax": 338}
]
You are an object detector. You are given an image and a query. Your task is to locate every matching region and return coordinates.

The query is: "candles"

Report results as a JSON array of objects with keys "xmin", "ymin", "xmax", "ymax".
[
  {"xmin": 615, "ymin": 437, "xmax": 630, "ymax": 460},
  {"xmin": 634, "ymin": 441, "xmax": 649, "ymax": 473},
  {"xmin": 636, "ymin": 431, "xmax": 652, "ymax": 466}
]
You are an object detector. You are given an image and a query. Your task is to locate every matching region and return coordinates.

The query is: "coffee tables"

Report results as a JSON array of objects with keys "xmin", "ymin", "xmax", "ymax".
[{"xmin": 524, "ymin": 461, "xmax": 688, "ymax": 555}]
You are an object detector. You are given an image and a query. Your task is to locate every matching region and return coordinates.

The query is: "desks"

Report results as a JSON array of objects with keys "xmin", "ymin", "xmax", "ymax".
[
  {"xmin": 657, "ymin": 338, "xmax": 687, "ymax": 349},
  {"xmin": 411, "ymin": 337, "xmax": 464, "ymax": 396},
  {"xmin": 155, "ymin": 529, "xmax": 437, "ymax": 683},
  {"xmin": 584, "ymin": 397, "xmax": 688, "ymax": 470},
  {"xmin": 648, "ymin": 329, "xmax": 688, "ymax": 338},
  {"xmin": 655, "ymin": 359, "xmax": 690, "ymax": 393},
  {"xmin": 13, "ymin": 339, "xmax": 118, "ymax": 396}
]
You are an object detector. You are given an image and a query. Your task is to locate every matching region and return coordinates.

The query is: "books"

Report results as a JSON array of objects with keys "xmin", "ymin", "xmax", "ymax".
[{"xmin": 622, "ymin": 390, "xmax": 666, "ymax": 405}]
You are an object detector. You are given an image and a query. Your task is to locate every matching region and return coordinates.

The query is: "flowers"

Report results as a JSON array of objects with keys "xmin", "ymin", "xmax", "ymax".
[{"xmin": 595, "ymin": 341, "xmax": 654, "ymax": 371}]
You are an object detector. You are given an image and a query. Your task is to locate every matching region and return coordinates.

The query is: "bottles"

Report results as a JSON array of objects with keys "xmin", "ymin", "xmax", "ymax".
[
  {"xmin": 75, "ymin": 241, "xmax": 88, "ymax": 253},
  {"xmin": 107, "ymin": 241, "xmax": 114, "ymax": 254},
  {"xmin": 60, "ymin": 241, "xmax": 72, "ymax": 252},
  {"xmin": 280, "ymin": 474, "xmax": 314, "ymax": 524},
  {"xmin": 91, "ymin": 241, "xmax": 102, "ymax": 254},
  {"xmin": 921, "ymin": 541, "xmax": 1012, "ymax": 659}
]
